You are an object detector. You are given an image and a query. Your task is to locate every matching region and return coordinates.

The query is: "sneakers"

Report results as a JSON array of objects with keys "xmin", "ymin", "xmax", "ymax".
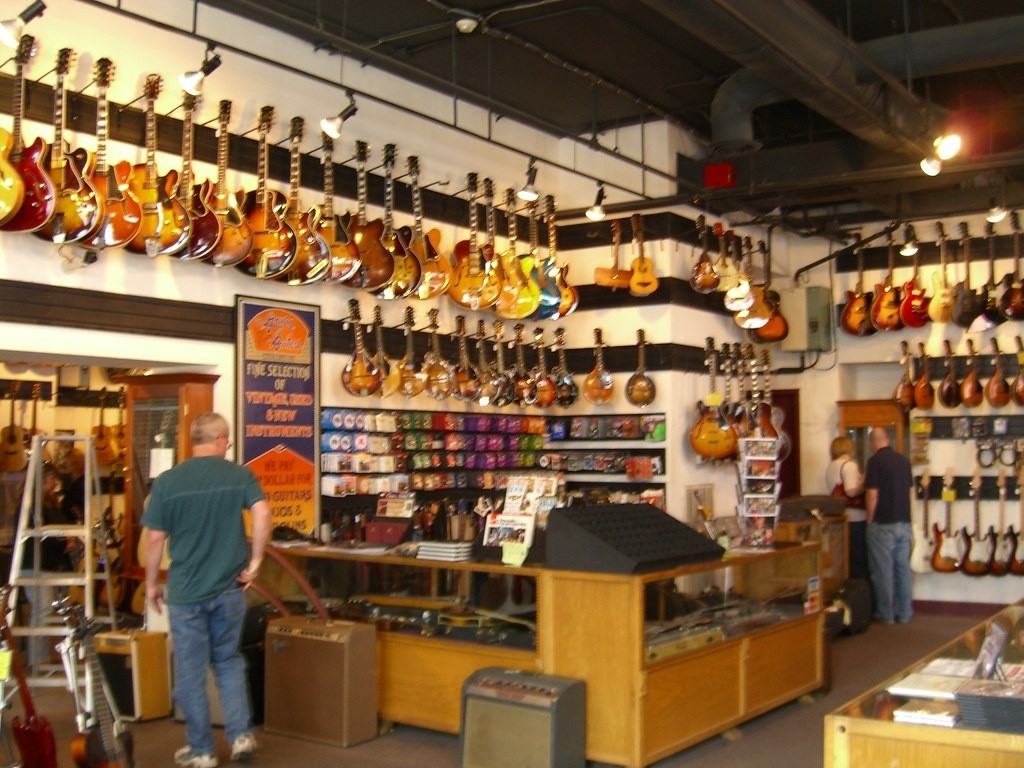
[
  {"xmin": 174, "ymin": 745, "xmax": 218, "ymax": 767},
  {"xmin": 230, "ymin": 731, "xmax": 259, "ymax": 763}
]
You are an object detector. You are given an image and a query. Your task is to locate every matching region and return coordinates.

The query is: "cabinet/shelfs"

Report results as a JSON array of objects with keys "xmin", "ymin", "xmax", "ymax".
[
  {"xmin": 546, "ymin": 414, "xmax": 668, "ymax": 483},
  {"xmin": 114, "ymin": 372, "xmax": 221, "ymax": 628},
  {"xmin": 838, "ymin": 398, "xmax": 909, "ymax": 497},
  {"xmin": 774, "ymin": 514, "xmax": 850, "ymax": 597}
]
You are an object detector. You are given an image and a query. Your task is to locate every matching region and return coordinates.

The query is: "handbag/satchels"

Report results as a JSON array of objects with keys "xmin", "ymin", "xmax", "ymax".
[{"xmin": 831, "ymin": 484, "xmax": 869, "ymax": 509}]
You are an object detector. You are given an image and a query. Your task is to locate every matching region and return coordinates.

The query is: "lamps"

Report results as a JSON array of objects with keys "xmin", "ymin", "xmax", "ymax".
[
  {"xmin": 179, "ymin": 43, "xmax": 222, "ymax": 95},
  {"xmin": 920, "ymin": 158, "xmax": 941, "ymax": 176},
  {"xmin": 898, "ymin": 226, "xmax": 918, "ymax": 257},
  {"xmin": 584, "ymin": 182, "xmax": 606, "ymax": 221},
  {"xmin": 321, "ymin": 90, "xmax": 357, "ymax": 139},
  {"xmin": 0, "ymin": 0, "xmax": 46, "ymax": 49},
  {"xmin": 516, "ymin": 159, "xmax": 540, "ymax": 201},
  {"xmin": 933, "ymin": 134, "xmax": 962, "ymax": 160},
  {"xmin": 985, "ymin": 186, "xmax": 1007, "ymax": 224}
]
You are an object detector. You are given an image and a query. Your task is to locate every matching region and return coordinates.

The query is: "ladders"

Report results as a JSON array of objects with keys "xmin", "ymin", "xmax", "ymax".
[{"xmin": 0, "ymin": 432, "xmax": 116, "ymax": 735}]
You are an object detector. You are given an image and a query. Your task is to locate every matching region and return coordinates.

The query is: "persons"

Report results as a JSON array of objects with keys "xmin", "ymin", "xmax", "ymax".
[
  {"xmin": 137, "ymin": 412, "xmax": 273, "ymax": 768},
  {"xmin": 825, "ymin": 436, "xmax": 867, "ymax": 577},
  {"xmin": 17, "ymin": 466, "xmax": 76, "ymax": 575},
  {"xmin": 865, "ymin": 427, "xmax": 914, "ymax": 626}
]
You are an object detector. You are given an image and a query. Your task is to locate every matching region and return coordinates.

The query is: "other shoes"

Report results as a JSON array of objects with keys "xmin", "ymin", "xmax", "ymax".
[{"xmin": 878, "ymin": 614, "xmax": 908, "ymax": 625}]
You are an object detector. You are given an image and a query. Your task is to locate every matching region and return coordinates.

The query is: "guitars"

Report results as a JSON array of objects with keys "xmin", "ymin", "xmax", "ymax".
[
  {"xmin": 0, "ymin": 582, "xmax": 60, "ymax": 768},
  {"xmin": 49, "ymin": 594, "xmax": 135, "ymax": 768},
  {"xmin": 0, "ymin": 30, "xmax": 1024, "ymax": 609}
]
